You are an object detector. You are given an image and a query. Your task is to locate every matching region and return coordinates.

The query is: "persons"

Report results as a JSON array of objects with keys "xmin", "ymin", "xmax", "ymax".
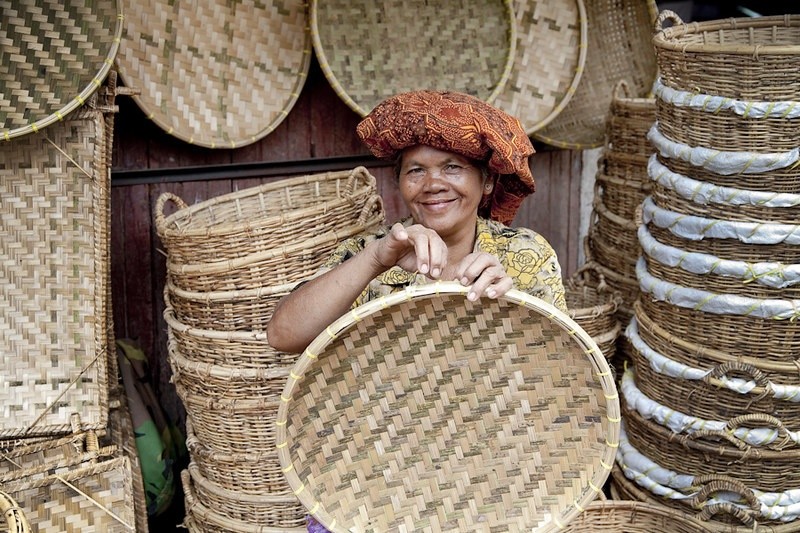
[{"xmin": 267, "ymin": 90, "xmax": 568, "ymax": 533}]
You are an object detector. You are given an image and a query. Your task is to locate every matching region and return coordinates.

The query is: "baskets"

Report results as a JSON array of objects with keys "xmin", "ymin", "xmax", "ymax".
[
  {"xmin": 153, "ymin": 166, "xmax": 389, "ymax": 533},
  {"xmin": 556, "ymin": 499, "xmax": 722, "ymax": 533},
  {"xmin": 488, "ymin": 0, "xmax": 588, "ymax": 134},
  {"xmin": 531, "ymin": 0, "xmax": 666, "ymax": 151},
  {"xmin": 0, "ymin": 0, "xmax": 124, "ymax": 140},
  {"xmin": 115, "ymin": 0, "xmax": 311, "ymax": 149},
  {"xmin": 565, "ymin": 9, "xmax": 800, "ymax": 533},
  {"xmin": 275, "ymin": 279, "xmax": 622, "ymax": 533},
  {"xmin": 312, "ymin": 0, "xmax": 517, "ymax": 121}
]
